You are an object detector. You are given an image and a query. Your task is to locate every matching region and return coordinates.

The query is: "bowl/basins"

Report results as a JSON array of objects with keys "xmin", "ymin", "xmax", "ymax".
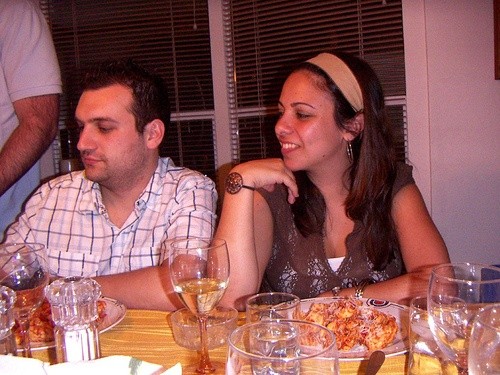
[{"xmin": 170, "ymin": 304, "xmax": 239, "ymax": 351}]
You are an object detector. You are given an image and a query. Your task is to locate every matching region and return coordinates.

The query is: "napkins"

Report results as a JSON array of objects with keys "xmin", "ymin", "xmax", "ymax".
[{"xmin": 0, "ymin": 355, "xmax": 183, "ymax": 375}]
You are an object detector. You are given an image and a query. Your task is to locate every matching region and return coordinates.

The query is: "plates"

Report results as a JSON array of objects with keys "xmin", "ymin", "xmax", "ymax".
[
  {"xmin": 268, "ymin": 295, "xmax": 420, "ymax": 362},
  {"xmin": 16, "ymin": 295, "xmax": 127, "ymax": 352}
]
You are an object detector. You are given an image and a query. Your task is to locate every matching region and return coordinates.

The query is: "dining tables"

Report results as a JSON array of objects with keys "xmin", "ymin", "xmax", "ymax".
[{"xmin": 17, "ymin": 309, "xmax": 470, "ymax": 375}]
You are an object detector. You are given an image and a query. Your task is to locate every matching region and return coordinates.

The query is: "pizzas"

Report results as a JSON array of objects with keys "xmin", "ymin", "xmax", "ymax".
[
  {"xmin": 295, "ymin": 303, "xmax": 398, "ymax": 351},
  {"xmin": 2, "ymin": 286, "xmax": 105, "ymax": 345}
]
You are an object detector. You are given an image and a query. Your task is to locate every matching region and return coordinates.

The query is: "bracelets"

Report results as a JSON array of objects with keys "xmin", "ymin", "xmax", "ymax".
[{"xmin": 224, "ymin": 172, "xmax": 255, "ymax": 195}]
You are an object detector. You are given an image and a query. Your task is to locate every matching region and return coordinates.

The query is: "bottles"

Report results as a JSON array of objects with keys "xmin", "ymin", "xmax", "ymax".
[{"xmin": 45, "ymin": 275, "xmax": 103, "ymax": 364}]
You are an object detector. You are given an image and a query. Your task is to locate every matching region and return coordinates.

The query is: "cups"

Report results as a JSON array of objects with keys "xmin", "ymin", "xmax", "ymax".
[
  {"xmin": 0, "ymin": 285, "xmax": 18, "ymax": 341},
  {"xmin": 407, "ymin": 261, "xmax": 500, "ymax": 375},
  {"xmin": 245, "ymin": 293, "xmax": 301, "ymax": 375},
  {"xmin": 224, "ymin": 317, "xmax": 341, "ymax": 375}
]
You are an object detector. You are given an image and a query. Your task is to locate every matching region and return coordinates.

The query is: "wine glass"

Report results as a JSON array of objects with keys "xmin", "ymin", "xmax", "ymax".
[
  {"xmin": 0, "ymin": 242, "xmax": 49, "ymax": 359},
  {"xmin": 168, "ymin": 237, "xmax": 231, "ymax": 375}
]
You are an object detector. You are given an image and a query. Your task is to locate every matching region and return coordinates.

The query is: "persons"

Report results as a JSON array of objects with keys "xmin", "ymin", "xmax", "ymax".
[
  {"xmin": 0, "ymin": 0, "xmax": 62, "ymax": 241},
  {"xmin": 205, "ymin": 50, "xmax": 458, "ymax": 310},
  {"xmin": 0, "ymin": 63, "xmax": 218, "ymax": 311}
]
